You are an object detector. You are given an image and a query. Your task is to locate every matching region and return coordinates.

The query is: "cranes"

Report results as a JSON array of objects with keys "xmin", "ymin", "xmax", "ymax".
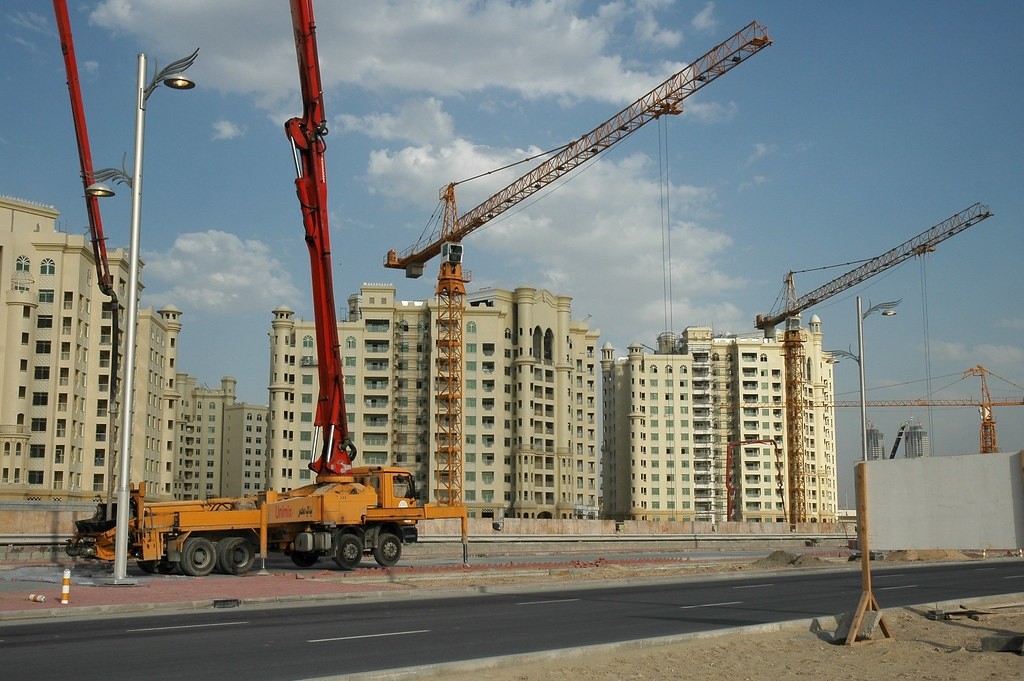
[
  {"xmin": 753, "ymin": 200, "xmax": 994, "ymax": 522},
  {"xmin": 799, "ymin": 359, "xmax": 1022, "ymax": 453},
  {"xmin": 382, "ymin": 16, "xmax": 780, "ymax": 519}
]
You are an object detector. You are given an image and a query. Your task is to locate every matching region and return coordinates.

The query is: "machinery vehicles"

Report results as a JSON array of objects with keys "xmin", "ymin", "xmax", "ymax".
[{"xmin": 63, "ymin": 0, "xmax": 472, "ymax": 575}]
